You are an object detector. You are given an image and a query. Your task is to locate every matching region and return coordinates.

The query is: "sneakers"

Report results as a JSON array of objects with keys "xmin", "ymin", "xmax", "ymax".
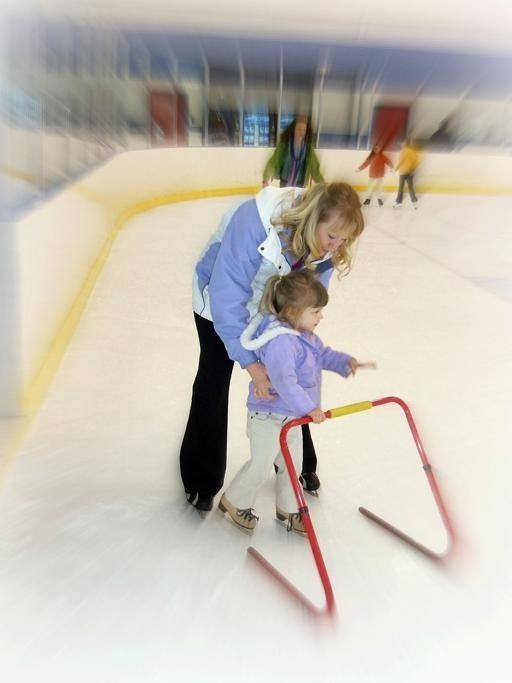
[
  {"xmin": 186, "ymin": 492, "xmax": 214, "ymax": 511},
  {"xmin": 275, "ymin": 506, "xmax": 307, "ymax": 532},
  {"xmin": 363, "ymin": 198, "xmax": 418, "ymax": 208},
  {"xmin": 218, "ymin": 493, "xmax": 257, "ymax": 529},
  {"xmin": 299, "ymin": 473, "xmax": 320, "ymax": 491}
]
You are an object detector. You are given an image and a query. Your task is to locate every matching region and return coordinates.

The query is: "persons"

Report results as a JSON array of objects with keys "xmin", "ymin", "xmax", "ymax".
[
  {"xmin": 429, "ymin": 120, "xmax": 454, "ymax": 152},
  {"xmin": 180, "ymin": 179, "xmax": 368, "ymax": 516},
  {"xmin": 393, "ymin": 135, "xmax": 419, "ymax": 209},
  {"xmin": 259, "ymin": 112, "xmax": 326, "ymax": 188},
  {"xmin": 216, "ymin": 271, "xmax": 360, "ymax": 535},
  {"xmin": 356, "ymin": 143, "xmax": 394, "ymax": 206}
]
